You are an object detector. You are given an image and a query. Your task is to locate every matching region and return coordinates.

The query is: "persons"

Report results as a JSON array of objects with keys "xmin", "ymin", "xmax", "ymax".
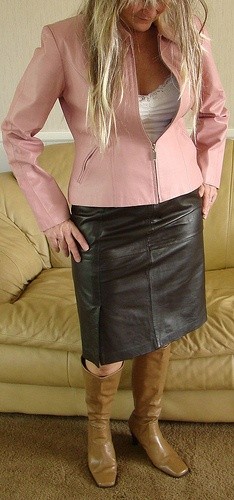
[{"xmin": 2, "ymin": 0, "xmax": 230, "ymax": 487}]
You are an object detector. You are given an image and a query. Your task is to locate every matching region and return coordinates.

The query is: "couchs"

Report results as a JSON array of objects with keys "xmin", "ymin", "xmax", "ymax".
[{"xmin": 0, "ymin": 136, "xmax": 234, "ymax": 425}]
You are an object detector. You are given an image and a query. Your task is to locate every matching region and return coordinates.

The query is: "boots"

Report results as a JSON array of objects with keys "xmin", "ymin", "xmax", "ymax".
[
  {"xmin": 80, "ymin": 354, "xmax": 125, "ymax": 488},
  {"xmin": 129, "ymin": 342, "xmax": 188, "ymax": 478}
]
